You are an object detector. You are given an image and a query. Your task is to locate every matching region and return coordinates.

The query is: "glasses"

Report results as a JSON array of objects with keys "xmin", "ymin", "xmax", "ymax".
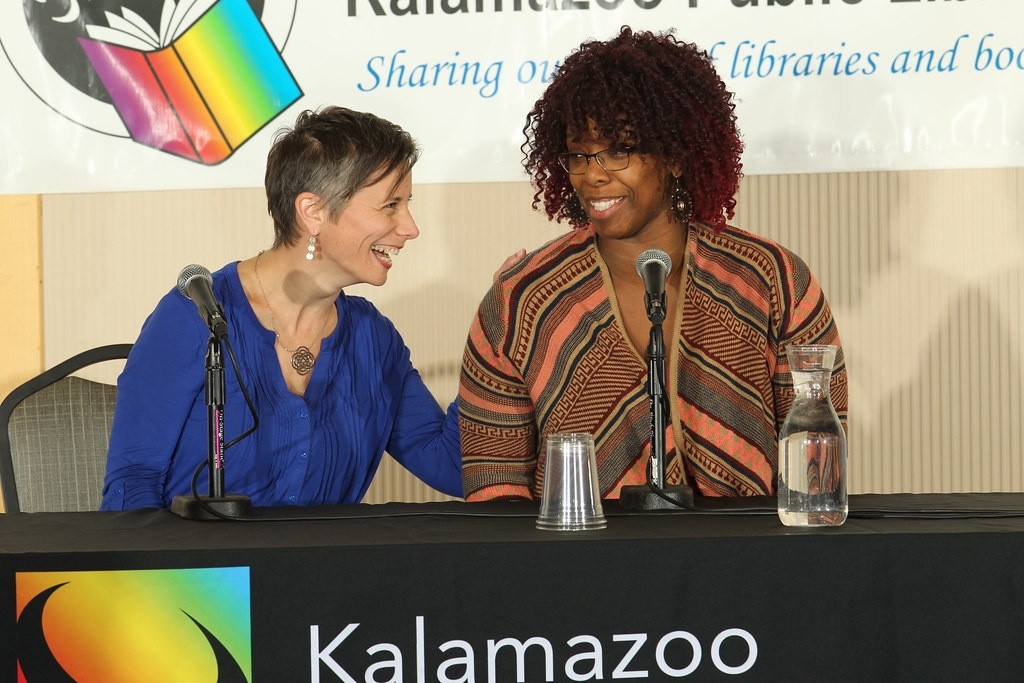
[{"xmin": 555, "ymin": 146, "xmax": 641, "ymax": 175}]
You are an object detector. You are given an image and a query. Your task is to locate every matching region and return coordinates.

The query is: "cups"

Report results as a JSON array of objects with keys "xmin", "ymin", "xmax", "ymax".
[{"xmin": 538, "ymin": 433, "xmax": 607, "ymax": 530}]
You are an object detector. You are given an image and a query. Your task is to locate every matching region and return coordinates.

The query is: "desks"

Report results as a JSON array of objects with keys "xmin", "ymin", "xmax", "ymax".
[{"xmin": 0, "ymin": 490, "xmax": 1024, "ymax": 683}]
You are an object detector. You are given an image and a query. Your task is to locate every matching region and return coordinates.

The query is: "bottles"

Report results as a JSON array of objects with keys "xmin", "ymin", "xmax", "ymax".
[{"xmin": 777, "ymin": 345, "xmax": 848, "ymax": 528}]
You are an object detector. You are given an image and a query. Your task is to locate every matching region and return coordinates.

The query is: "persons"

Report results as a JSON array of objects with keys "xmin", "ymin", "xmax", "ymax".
[
  {"xmin": 99, "ymin": 109, "xmax": 526, "ymax": 513},
  {"xmin": 459, "ymin": 32, "xmax": 849, "ymax": 502}
]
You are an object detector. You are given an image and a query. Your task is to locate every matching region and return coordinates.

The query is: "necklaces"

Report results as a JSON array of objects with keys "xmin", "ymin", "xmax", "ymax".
[{"xmin": 254, "ymin": 250, "xmax": 334, "ymax": 375}]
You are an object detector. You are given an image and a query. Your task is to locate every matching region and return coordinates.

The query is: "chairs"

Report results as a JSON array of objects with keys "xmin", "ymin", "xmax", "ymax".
[{"xmin": 0, "ymin": 341, "xmax": 137, "ymax": 514}]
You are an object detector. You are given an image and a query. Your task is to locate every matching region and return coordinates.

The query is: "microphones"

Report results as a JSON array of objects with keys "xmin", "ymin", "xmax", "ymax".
[
  {"xmin": 176, "ymin": 263, "xmax": 228, "ymax": 339},
  {"xmin": 636, "ymin": 248, "xmax": 672, "ymax": 325}
]
